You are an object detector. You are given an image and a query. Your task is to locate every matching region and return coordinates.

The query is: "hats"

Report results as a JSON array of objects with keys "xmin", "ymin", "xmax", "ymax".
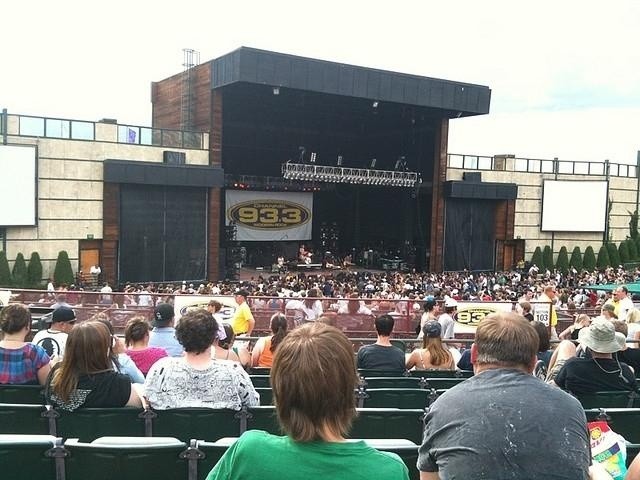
[
  {"xmin": 52, "ymin": 306, "xmax": 77, "ymax": 322},
  {"xmin": 578, "ymin": 316, "xmax": 626, "ymax": 354},
  {"xmin": 154, "ymin": 304, "xmax": 174, "ymax": 327},
  {"xmin": 445, "ymin": 299, "xmax": 458, "ymax": 308},
  {"xmin": 423, "ymin": 320, "xmax": 441, "ymax": 337},
  {"xmin": 234, "ymin": 290, "xmax": 249, "ymax": 295}
]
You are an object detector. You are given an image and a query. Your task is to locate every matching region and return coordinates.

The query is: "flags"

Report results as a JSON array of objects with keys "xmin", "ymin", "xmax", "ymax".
[{"xmin": 129, "ymin": 128, "xmax": 136, "ymax": 143}]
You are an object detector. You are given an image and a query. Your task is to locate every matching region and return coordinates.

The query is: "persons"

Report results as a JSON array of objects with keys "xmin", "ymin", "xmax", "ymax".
[
  {"xmin": 416, "ymin": 310, "xmax": 592, "ymax": 480},
  {"xmin": 1, "ymin": 242, "xmax": 639, "ymax": 412},
  {"xmin": 206, "ymin": 321, "xmax": 409, "ymax": 479},
  {"xmin": 624, "ymin": 452, "xmax": 640, "ymax": 480}
]
